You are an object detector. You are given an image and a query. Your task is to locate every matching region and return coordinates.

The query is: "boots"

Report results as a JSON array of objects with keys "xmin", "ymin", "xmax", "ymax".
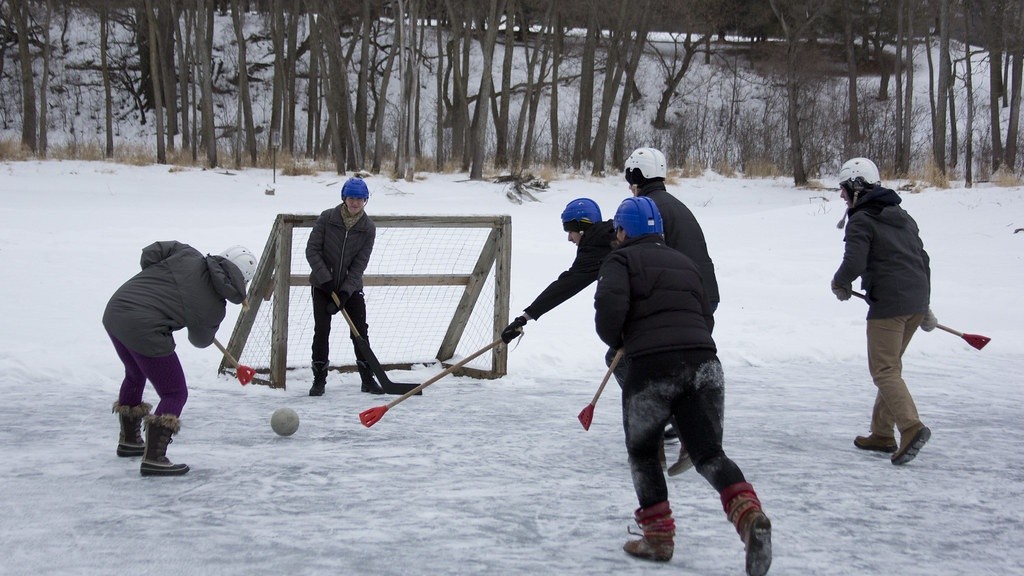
[
  {"xmin": 113, "ymin": 399, "xmax": 148, "ymax": 456},
  {"xmin": 623, "ymin": 500, "xmax": 676, "ymax": 561},
  {"xmin": 356, "ymin": 360, "xmax": 385, "ymax": 394},
  {"xmin": 309, "ymin": 359, "xmax": 330, "ymax": 396},
  {"xmin": 721, "ymin": 484, "xmax": 772, "ymax": 576},
  {"xmin": 139, "ymin": 413, "xmax": 189, "ymax": 476}
]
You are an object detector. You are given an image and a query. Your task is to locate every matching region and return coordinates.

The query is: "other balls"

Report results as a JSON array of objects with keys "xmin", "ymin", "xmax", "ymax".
[{"xmin": 270, "ymin": 408, "xmax": 300, "ymax": 437}]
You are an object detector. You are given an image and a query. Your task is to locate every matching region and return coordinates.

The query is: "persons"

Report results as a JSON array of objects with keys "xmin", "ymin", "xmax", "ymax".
[
  {"xmin": 102, "ymin": 241, "xmax": 257, "ymax": 476},
  {"xmin": 595, "ymin": 196, "xmax": 772, "ymax": 576},
  {"xmin": 831, "ymin": 158, "xmax": 937, "ymax": 465},
  {"xmin": 624, "ymin": 148, "xmax": 720, "ymax": 443},
  {"xmin": 501, "ymin": 198, "xmax": 695, "ymax": 476},
  {"xmin": 306, "ymin": 177, "xmax": 385, "ymax": 396}
]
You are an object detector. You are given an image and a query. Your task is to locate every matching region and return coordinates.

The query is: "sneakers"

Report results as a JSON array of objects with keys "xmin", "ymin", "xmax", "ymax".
[
  {"xmin": 853, "ymin": 433, "xmax": 897, "ymax": 452},
  {"xmin": 892, "ymin": 420, "xmax": 931, "ymax": 465}
]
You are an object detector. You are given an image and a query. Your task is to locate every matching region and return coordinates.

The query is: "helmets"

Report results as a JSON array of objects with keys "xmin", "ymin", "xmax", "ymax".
[
  {"xmin": 561, "ymin": 198, "xmax": 602, "ymax": 225},
  {"xmin": 341, "ymin": 177, "xmax": 368, "ymax": 199},
  {"xmin": 838, "ymin": 157, "xmax": 881, "ymax": 186},
  {"xmin": 218, "ymin": 246, "xmax": 258, "ymax": 282},
  {"xmin": 623, "ymin": 145, "xmax": 666, "ymax": 182},
  {"xmin": 612, "ymin": 196, "xmax": 663, "ymax": 239}
]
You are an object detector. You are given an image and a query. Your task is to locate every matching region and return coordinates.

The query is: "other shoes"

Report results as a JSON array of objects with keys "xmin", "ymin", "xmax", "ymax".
[
  {"xmin": 663, "ymin": 427, "xmax": 680, "ymax": 444},
  {"xmin": 667, "ymin": 445, "xmax": 695, "ymax": 477}
]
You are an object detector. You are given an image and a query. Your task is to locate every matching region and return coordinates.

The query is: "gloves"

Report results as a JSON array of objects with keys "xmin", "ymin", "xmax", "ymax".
[
  {"xmin": 326, "ymin": 289, "xmax": 348, "ymax": 315},
  {"xmin": 920, "ymin": 309, "xmax": 937, "ymax": 332},
  {"xmin": 501, "ymin": 316, "xmax": 529, "ymax": 347},
  {"xmin": 831, "ymin": 277, "xmax": 854, "ymax": 300},
  {"xmin": 322, "ymin": 280, "xmax": 339, "ymax": 304}
]
